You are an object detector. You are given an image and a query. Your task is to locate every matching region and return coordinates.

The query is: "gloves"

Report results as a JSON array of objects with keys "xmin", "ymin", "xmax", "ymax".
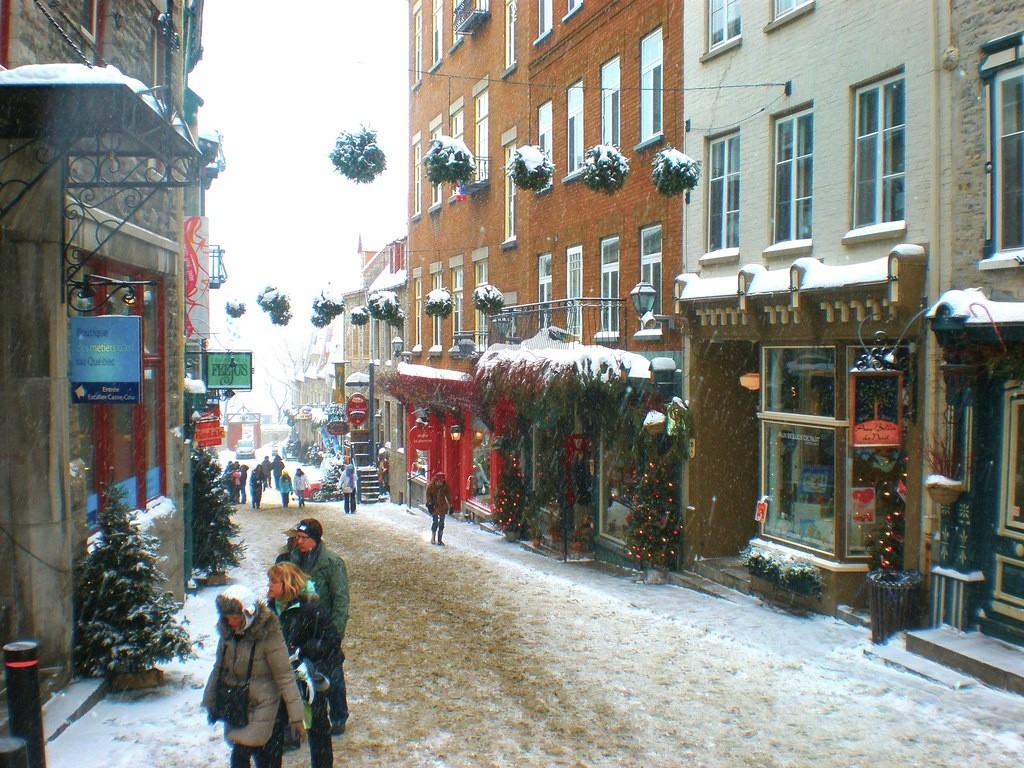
[
  {"xmin": 449, "ymin": 507, "xmax": 454, "ymax": 514},
  {"xmin": 428, "ymin": 504, "xmax": 434, "ymax": 514}
]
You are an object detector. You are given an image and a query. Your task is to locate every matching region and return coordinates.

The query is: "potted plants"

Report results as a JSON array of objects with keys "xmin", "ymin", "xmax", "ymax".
[{"xmin": 910, "ymin": 429, "xmax": 970, "ymax": 504}]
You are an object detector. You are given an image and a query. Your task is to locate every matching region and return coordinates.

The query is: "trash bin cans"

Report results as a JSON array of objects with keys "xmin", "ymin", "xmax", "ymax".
[{"xmin": 866, "ymin": 571, "xmax": 924, "ymax": 644}]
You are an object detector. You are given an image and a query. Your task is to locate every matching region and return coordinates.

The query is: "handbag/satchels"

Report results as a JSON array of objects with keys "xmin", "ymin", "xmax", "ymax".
[
  {"xmin": 211, "ymin": 679, "xmax": 250, "ymax": 726},
  {"xmin": 344, "ymin": 486, "xmax": 352, "ymax": 493}
]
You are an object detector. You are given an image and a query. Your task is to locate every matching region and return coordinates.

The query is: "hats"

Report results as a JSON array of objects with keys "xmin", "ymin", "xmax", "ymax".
[
  {"xmin": 282, "ymin": 468, "xmax": 289, "ymax": 475},
  {"xmin": 215, "ymin": 586, "xmax": 259, "ymax": 636},
  {"xmin": 296, "ymin": 518, "xmax": 322, "ymax": 545},
  {"xmin": 347, "ymin": 464, "xmax": 353, "ymax": 470},
  {"xmin": 282, "ymin": 523, "xmax": 301, "ymax": 539},
  {"xmin": 436, "ymin": 472, "xmax": 446, "ymax": 480}
]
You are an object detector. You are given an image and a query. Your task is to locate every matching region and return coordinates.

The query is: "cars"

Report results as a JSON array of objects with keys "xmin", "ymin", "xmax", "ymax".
[
  {"xmin": 235, "ymin": 439, "xmax": 256, "ymax": 459},
  {"xmin": 294, "ymin": 478, "xmax": 326, "ymax": 502}
]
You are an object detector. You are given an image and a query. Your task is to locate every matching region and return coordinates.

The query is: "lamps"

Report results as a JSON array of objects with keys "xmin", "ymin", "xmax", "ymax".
[
  {"xmin": 629, "ymin": 279, "xmax": 676, "ymax": 333},
  {"xmin": 392, "ymin": 334, "xmax": 405, "ymax": 355},
  {"xmin": 451, "ymin": 424, "xmax": 465, "ymax": 442},
  {"xmin": 490, "ymin": 316, "xmax": 520, "ymax": 345}
]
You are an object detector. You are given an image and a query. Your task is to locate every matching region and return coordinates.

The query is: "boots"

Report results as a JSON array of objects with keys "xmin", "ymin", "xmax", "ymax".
[
  {"xmin": 431, "ymin": 531, "xmax": 436, "ymax": 543},
  {"xmin": 437, "ymin": 531, "xmax": 444, "ymax": 545}
]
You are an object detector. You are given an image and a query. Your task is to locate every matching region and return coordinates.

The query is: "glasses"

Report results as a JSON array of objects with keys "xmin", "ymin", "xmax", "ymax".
[{"xmin": 296, "ymin": 534, "xmax": 310, "ymax": 541}]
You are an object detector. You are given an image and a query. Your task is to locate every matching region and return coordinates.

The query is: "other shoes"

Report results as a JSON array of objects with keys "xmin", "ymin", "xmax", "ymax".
[
  {"xmin": 351, "ymin": 510, "xmax": 357, "ymax": 514},
  {"xmin": 330, "ymin": 720, "xmax": 345, "ymax": 735},
  {"xmin": 283, "ymin": 737, "xmax": 300, "ymax": 752}
]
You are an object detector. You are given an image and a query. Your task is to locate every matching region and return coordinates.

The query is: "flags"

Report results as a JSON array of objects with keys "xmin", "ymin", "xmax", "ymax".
[{"xmin": 456, "ymin": 182, "xmax": 466, "ymax": 201}]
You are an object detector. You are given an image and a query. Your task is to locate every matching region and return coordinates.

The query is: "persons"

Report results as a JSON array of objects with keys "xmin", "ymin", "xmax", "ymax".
[
  {"xmin": 222, "ymin": 460, "xmax": 249, "ymax": 504},
  {"xmin": 426, "ymin": 472, "xmax": 450, "ymax": 546},
  {"xmin": 339, "ymin": 464, "xmax": 358, "ymax": 513},
  {"xmin": 294, "ymin": 468, "xmax": 308, "ymax": 507},
  {"xmin": 249, "ymin": 464, "xmax": 266, "ymax": 509},
  {"xmin": 278, "ymin": 468, "xmax": 293, "ymax": 506},
  {"xmin": 202, "ymin": 584, "xmax": 306, "ymax": 768},
  {"xmin": 274, "ymin": 518, "xmax": 350, "ymax": 735},
  {"xmin": 261, "ymin": 561, "xmax": 337, "ymax": 768},
  {"xmin": 261, "ymin": 453, "xmax": 285, "ymax": 492}
]
